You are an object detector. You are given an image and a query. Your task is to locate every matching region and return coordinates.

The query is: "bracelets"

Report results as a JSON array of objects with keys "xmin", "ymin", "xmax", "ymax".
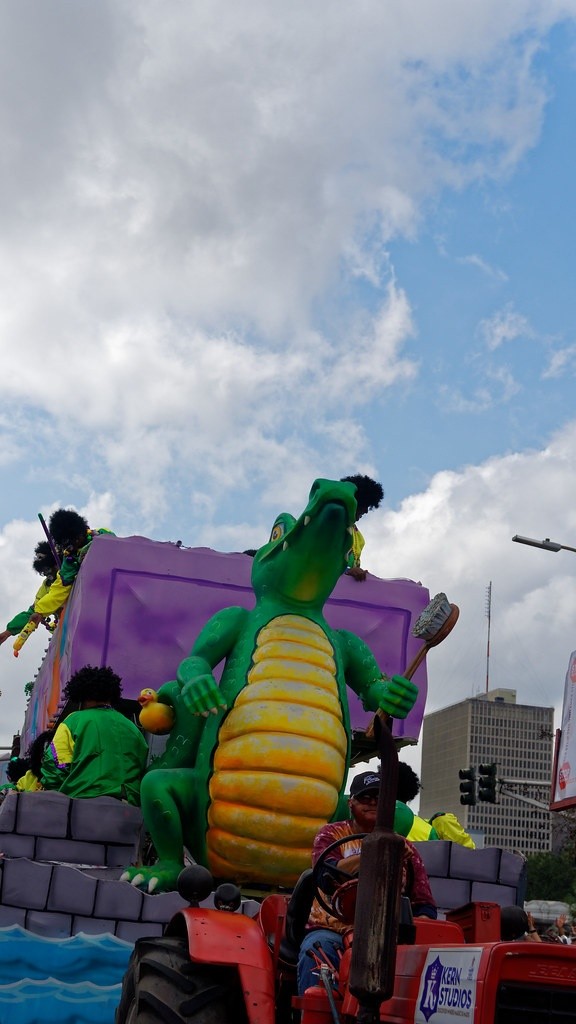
[{"xmin": 530, "ymin": 929, "xmax": 537, "ymax": 933}]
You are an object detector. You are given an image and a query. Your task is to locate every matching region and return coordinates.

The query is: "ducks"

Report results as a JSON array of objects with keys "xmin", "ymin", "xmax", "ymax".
[{"xmin": 136, "ymin": 687, "xmax": 175, "ymax": 736}]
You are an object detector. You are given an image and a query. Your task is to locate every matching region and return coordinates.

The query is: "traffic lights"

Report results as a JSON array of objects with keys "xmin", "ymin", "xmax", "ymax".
[
  {"xmin": 457, "ymin": 767, "xmax": 477, "ymax": 805},
  {"xmin": 477, "ymin": 759, "xmax": 498, "ymax": 805}
]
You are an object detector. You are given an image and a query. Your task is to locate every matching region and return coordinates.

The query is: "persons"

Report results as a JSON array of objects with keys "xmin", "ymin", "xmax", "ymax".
[
  {"xmin": 296, "ymin": 775, "xmax": 438, "ymax": 1010},
  {"xmin": 0, "ymin": 664, "xmax": 149, "ymax": 807},
  {"xmin": 372, "ymin": 762, "xmax": 423, "ymax": 801},
  {"xmin": 0, "ymin": 510, "xmax": 118, "ymax": 644},
  {"xmin": 501, "ymin": 903, "xmax": 576, "ymax": 945}
]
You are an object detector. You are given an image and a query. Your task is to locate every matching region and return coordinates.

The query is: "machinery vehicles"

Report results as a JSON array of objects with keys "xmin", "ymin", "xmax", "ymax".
[{"xmin": 114, "ymin": 712, "xmax": 575, "ymax": 1024}]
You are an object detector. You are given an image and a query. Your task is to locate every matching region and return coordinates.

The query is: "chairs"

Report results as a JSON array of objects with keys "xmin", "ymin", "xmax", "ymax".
[{"xmin": 284, "ymin": 868, "xmax": 318, "ymax": 949}]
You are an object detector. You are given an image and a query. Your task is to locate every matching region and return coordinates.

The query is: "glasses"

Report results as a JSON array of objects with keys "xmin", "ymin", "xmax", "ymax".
[{"xmin": 353, "ymin": 795, "xmax": 378, "ymax": 804}]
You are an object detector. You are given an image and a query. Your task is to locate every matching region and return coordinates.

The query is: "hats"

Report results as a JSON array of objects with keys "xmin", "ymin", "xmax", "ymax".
[
  {"xmin": 499, "ymin": 905, "xmax": 528, "ymax": 940},
  {"xmin": 350, "ymin": 771, "xmax": 381, "ymax": 800}
]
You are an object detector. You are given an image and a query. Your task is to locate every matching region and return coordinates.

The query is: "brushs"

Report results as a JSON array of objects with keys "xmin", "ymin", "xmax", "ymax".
[{"xmin": 365, "ymin": 592, "xmax": 461, "ymax": 739}]
